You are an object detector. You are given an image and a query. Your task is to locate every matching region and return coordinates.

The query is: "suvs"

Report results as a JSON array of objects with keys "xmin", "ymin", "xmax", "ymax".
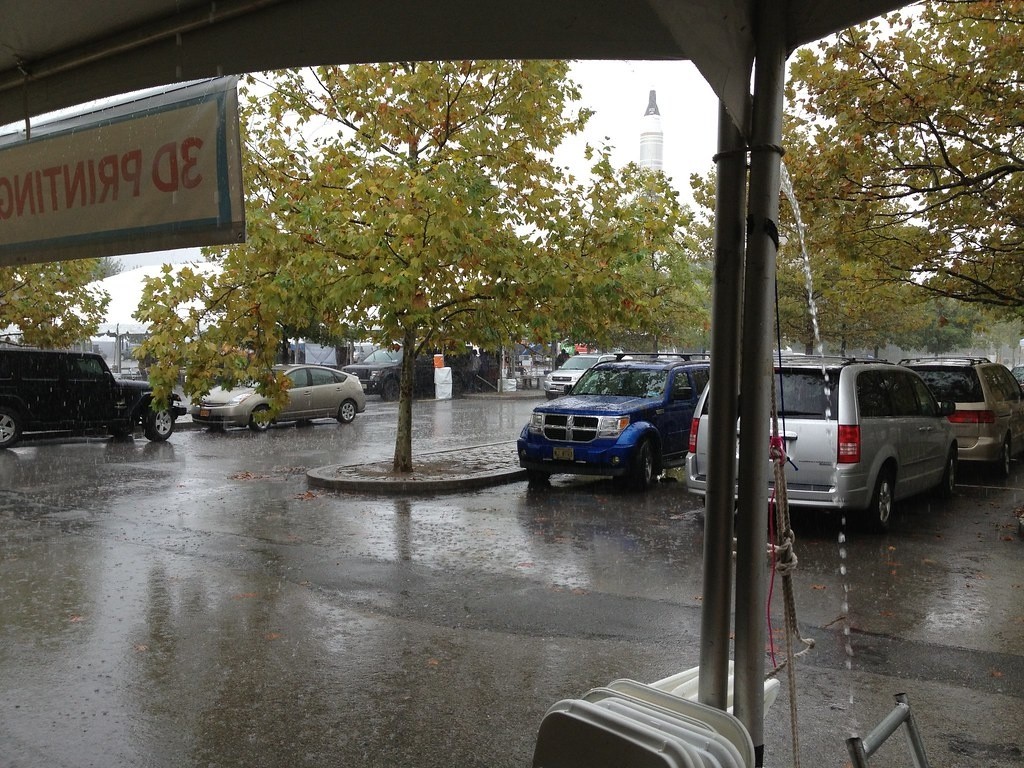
[
  {"xmin": 515, "ymin": 350, "xmax": 1024, "ymax": 532},
  {"xmin": 341, "ymin": 344, "xmax": 475, "ymax": 401},
  {"xmin": 0, "ymin": 340, "xmax": 187, "ymax": 449}
]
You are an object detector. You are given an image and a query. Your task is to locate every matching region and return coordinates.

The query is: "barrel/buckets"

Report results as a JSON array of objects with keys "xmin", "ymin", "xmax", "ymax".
[{"xmin": 434, "ymin": 354, "xmax": 444, "ymax": 368}]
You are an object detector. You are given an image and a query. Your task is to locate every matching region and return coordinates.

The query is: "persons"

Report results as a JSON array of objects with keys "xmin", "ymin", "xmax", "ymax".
[
  {"xmin": 556, "ymin": 349, "xmax": 580, "ymax": 370},
  {"xmin": 466, "ymin": 347, "xmax": 490, "ymax": 393}
]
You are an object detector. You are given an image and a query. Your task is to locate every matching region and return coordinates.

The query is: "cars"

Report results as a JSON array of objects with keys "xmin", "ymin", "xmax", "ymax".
[{"xmin": 187, "ymin": 363, "xmax": 366, "ymax": 431}]
